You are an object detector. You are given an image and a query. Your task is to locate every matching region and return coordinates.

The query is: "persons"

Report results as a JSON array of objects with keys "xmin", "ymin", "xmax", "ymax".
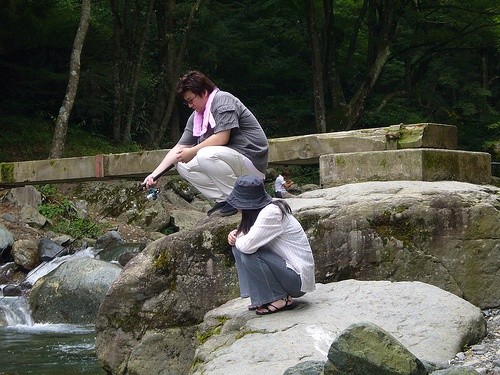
[
  {"xmin": 228, "ymin": 175, "xmax": 315, "ymax": 315},
  {"xmin": 275, "ymin": 171, "xmax": 297, "ymax": 198},
  {"xmin": 141, "ymin": 71, "xmax": 269, "ymax": 216}
]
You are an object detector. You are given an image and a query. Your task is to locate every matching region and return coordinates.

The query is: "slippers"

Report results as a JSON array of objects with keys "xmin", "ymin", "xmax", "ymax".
[
  {"xmin": 247, "ymin": 301, "xmax": 269, "ymax": 310},
  {"xmin": 256, "ymin": 296, "xmax": 292, "ymax": 314}
]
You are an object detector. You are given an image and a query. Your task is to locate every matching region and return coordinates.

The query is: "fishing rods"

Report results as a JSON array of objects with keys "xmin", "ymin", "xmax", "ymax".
[
  {"xmin": 0, "ymin": 163, "xmax": 176, "ymax": 301},
  {"xmin": 269, "ymin": 172, "xmax": 320, "ymax": 185}
]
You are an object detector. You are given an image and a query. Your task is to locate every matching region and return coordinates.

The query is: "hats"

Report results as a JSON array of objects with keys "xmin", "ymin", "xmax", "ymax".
[{"xmin": 225, "ymin": 174, "xmax": 272, "ymax": 210}]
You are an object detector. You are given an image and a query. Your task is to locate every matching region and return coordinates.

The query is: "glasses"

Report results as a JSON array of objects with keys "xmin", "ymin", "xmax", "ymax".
[{"xmin": 182, "ymin": 95, "xmax": 197, "ymax": 106}]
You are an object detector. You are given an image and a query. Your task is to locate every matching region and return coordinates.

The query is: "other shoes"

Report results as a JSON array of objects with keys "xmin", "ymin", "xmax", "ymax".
[
  {"xmin": 207, "ymin": 202, "xmax": 227, "ymax": 214},
  {"xmin": 220, "ymin": 203, "xmax": 238, "ymax": 217}
]
